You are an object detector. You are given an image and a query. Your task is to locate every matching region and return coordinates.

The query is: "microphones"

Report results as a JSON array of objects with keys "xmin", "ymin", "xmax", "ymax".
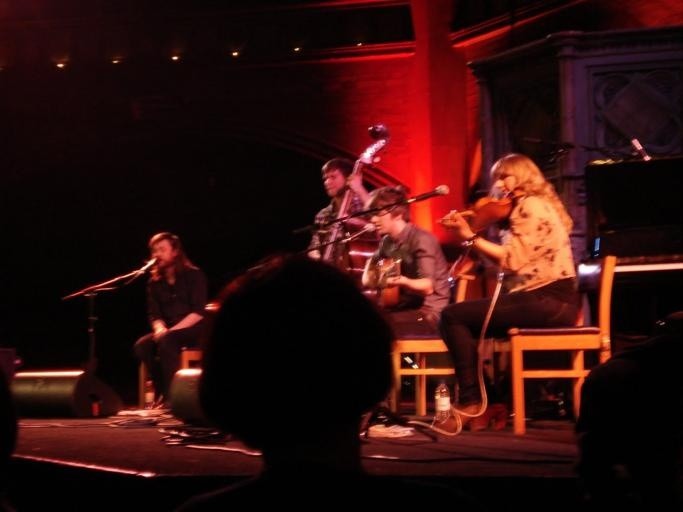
[
  {"xmin": 141, "ymin": 257, "xmax": 160, "ymax": 271},
  {"xmin": 342, "ymin": 223, "xmax": 375, "ymax": 244},
  {"xmin": 401, "ymin": 185, "xmax": 449, "ymax": 205}
]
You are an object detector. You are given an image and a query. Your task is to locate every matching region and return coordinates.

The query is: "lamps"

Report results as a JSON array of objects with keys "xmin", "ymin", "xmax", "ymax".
[
  {"xmin": 165, "ymin": 369, "xmax": 217, "ymax": 428},
  {"xmin": 7, "ymin": 368, "xmax": 124, "ymax": 420}
]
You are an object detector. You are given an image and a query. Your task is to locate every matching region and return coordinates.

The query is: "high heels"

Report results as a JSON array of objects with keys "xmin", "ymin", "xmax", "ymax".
[{"xmin": 431, "ymin": 403, "xmax": 490, "ymax": 436}]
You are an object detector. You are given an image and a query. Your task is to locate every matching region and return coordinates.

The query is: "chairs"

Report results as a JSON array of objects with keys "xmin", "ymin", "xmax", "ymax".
[
  {"xmin": 377, "ymin": 253, "xmax": 476, "ymax": 421},
  {"xmin": 489, "ymin": 251, "xmax": 618, "ymax": 438}
]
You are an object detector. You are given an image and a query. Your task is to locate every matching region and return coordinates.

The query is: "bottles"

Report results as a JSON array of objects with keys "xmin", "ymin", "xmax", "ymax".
[
  {"xmin": 143, "ymin": 380, "xmax": 156, "ymax": 408},
  {"xmin": 559, "ymin": 392, "xmax": 568, "ymax": 420},
  {"xmin": 434, "ymin": 379, "xmax": 453, "ymax": 423}
]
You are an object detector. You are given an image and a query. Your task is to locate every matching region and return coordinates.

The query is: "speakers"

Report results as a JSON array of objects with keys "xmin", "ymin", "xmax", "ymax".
[
  {"xmin": 169, "ymin": 368, "xmax": 202, "ymax": 425},
  {"xmin": 10, "ymin": 371, "xmax": 86, "ymax": 418},
  {"xmin": 578, "ymin": 269, "xmax": 683, "ymax": 366},
  {"xmin": 585, "ymin": 158, "xmax": 683, "ymax": 254}
]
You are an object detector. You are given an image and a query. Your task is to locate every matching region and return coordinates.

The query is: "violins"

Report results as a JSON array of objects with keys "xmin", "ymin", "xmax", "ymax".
[{"xmin": 441, "ymin": 198, "xmax": 512, "ymax": 237}]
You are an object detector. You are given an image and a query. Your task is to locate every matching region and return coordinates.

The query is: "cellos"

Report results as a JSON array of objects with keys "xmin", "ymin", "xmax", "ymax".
[{"xmin": 321, "ymin": 122, "xmax": 388, "ymax": 265}]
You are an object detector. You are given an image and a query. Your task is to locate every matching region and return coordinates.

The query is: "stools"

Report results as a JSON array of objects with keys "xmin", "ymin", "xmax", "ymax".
[{"xmin": 132, "ymin": 338, "xmax": 202, "ymax": 412}]
